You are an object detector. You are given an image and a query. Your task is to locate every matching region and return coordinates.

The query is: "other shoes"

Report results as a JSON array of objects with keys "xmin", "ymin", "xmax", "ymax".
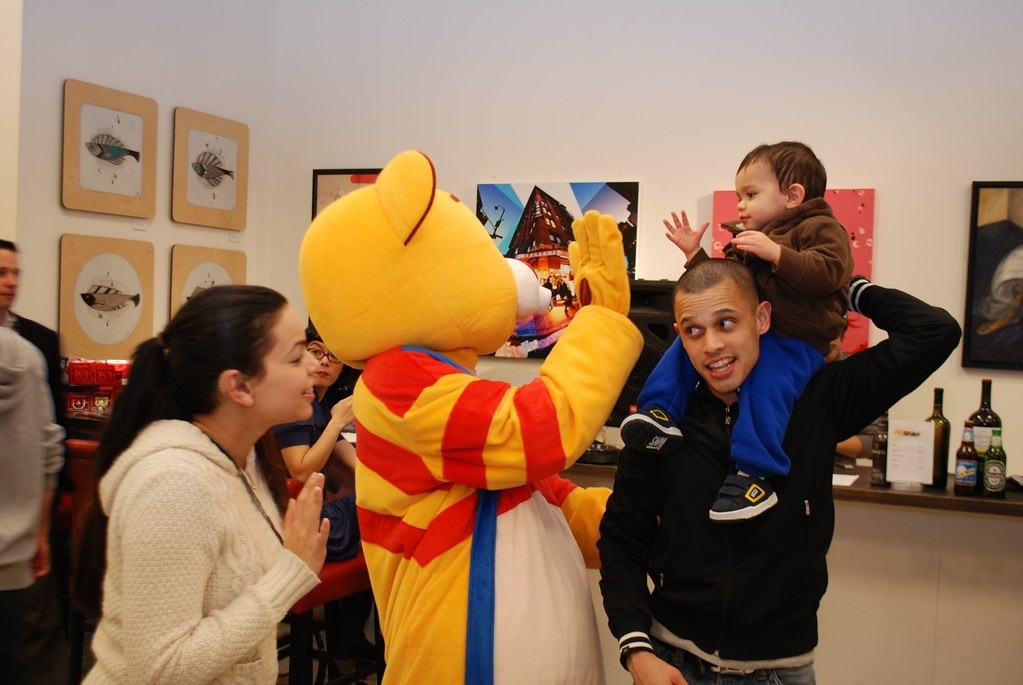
[{"xmin": 354, "ymin": 638, "xmax": 378, "ymax": 662}]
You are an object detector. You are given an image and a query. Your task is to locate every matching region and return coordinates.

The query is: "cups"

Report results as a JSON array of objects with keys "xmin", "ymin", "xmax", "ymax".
[{"xmin": 590, "ymin": 443, "xmax": 614, "ymax": 452}]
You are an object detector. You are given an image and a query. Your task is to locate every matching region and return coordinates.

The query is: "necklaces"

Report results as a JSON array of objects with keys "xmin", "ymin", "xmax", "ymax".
[{"xmin": 205, "ymin": 432, "xmax": 284, "ymax": 548}]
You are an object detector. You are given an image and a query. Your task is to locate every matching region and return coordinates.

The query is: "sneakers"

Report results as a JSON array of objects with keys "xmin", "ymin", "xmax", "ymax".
[
  {"xmin": 710, "ymin": 473, "xmax": 778, "ymax": 520},
  {"xmin": 619, "ymin": 405, "xmax": 684, "ymax": 455}
]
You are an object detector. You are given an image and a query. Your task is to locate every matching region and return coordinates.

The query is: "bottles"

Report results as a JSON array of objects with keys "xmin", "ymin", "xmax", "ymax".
[
  {"xmin": 952, "ymin": 419, "xmax": 979, "ymax": 495},
  {"xmin": 924, "ymin": 388, "xmax": 951, "ymax": 491},
  {"xmin": 969, "ymin": 378, "xmax": 1003, "ymax": 492},
  {"xmin": 981, "ymin": 427, "xmax": 1007, "ymax": 500},
  {"xmin": 871, "ymin": 410, "xmax": 892, "ymax": 487}
]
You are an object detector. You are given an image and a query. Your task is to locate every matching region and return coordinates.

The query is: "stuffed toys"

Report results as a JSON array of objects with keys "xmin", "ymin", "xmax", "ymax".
[{"xmin": 299, "ymin": 150, "xmax": 646, "ymax": 685}]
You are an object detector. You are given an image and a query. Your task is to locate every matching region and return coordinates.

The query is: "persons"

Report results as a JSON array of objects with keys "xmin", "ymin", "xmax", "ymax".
[
  {"xmin": 596, "ymin": 259, "xmax": 963, "ymax": 685},
  {"xmin": 0, "ymin": 237, "xmax": 379, "ymax": 685},
  {"xmin": 619, "ymin": 140, "xmax": 854, "ymax": 521}
]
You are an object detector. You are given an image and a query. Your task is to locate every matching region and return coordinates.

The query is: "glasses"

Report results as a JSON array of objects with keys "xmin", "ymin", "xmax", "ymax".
[{"xmin": 307, "ymin": 348, "xmax": 341, "ymax": 363}]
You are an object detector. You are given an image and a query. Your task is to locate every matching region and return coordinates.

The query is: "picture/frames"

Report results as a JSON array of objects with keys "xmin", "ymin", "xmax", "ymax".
[
  {"xmin": 170, "ymin": 106, "xmax": 250, "ymax": 230},
  {"xmin": 312, "ymin": 168, "xmax": 384, "ymax": 222},
  {"xmin": 170, "ymin": 244, "xmax": 247, "ymax": 323},
  {"xmin": 58, "ymin": 234, "xmax": 156, "ymax": 361},
  {"xmin": 962, "ymin": 180, "xmax": 1023, "ymax": 370},
  {"xmin": 60, "ymin": 79, "xmax": 159, "ymax": 218}
]
